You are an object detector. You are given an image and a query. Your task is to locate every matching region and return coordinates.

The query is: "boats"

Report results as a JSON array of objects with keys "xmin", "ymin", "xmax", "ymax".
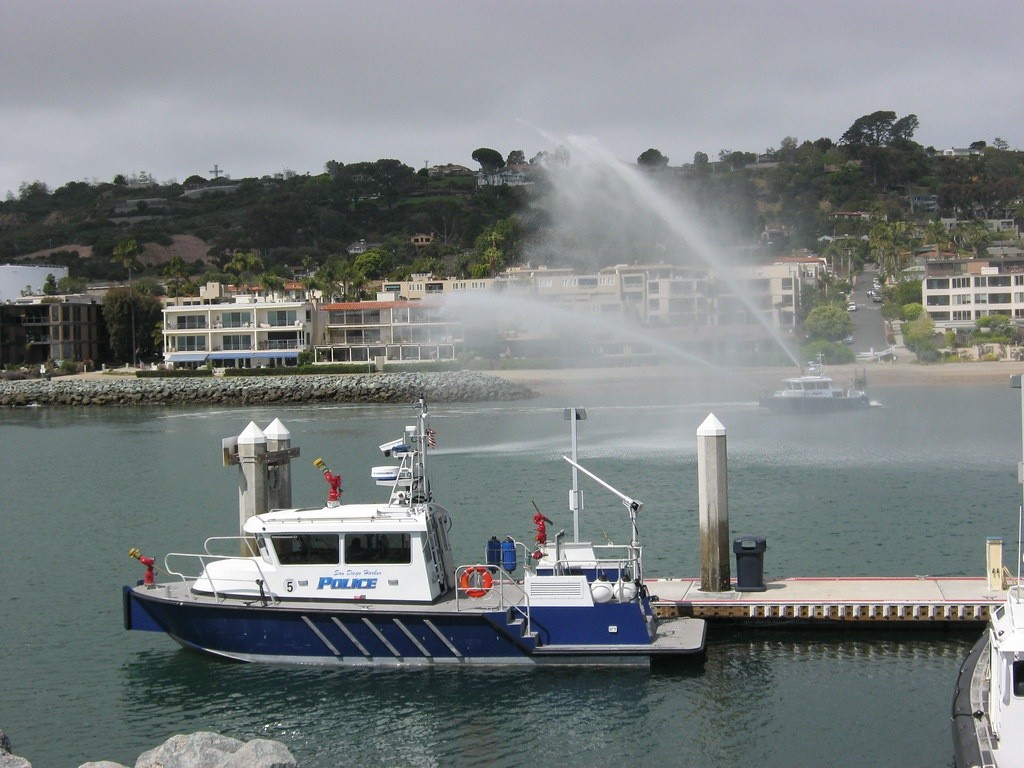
[
  {"xmin": 758, "ymin": 350, "xmax": 883, "ymax": 409},
  {"xmin": 951, "ymin": 583, "xmax": 1024, "ymax": 768},
  {"xmin": 121, "ymin": 392, "xmax": 708, "ymax": 670}
]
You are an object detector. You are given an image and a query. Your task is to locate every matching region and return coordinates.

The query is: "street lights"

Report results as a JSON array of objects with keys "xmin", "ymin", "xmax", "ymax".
[{"xmin": 831, "ymin": 292, "xmax": 845, "ymax": 310}]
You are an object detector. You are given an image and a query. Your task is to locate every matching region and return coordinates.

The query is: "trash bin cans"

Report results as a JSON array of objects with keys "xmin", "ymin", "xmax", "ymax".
[{"xmin": 732, "ymin": 537, "xmax": 767, "ymax": 593}]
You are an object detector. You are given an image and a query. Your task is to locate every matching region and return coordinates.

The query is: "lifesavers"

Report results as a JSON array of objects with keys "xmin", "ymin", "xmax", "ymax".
[{"xmin": 460, "ymin": 566, "xmax": 492, "ymax": 597}]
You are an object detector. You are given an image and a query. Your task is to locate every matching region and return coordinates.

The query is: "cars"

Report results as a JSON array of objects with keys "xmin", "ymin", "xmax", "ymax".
[
  {"xmin": 842, "ymin": 333, "xmax": 855, "ymax": 343},
  {"xmin": 872, "ymin": 277, "xmax": 879, "ymax": 284},
  {"xmin": 874, "ymin": 285, "xmax": 881, "ymax": 291},
  {"xmin": 866, "ymin": 289, "xmax": 878, "ymax": 298},
  {"xmin": 872, "ymin": 295, "xmax": 882, "ymax": 303},
  {"xmin": 847, "ymin": 302, "xmax": 857, "ymax": 311},
  {"xmin": 872, "ymin": 282, "xmax": 880, "ymax": 288}
]
